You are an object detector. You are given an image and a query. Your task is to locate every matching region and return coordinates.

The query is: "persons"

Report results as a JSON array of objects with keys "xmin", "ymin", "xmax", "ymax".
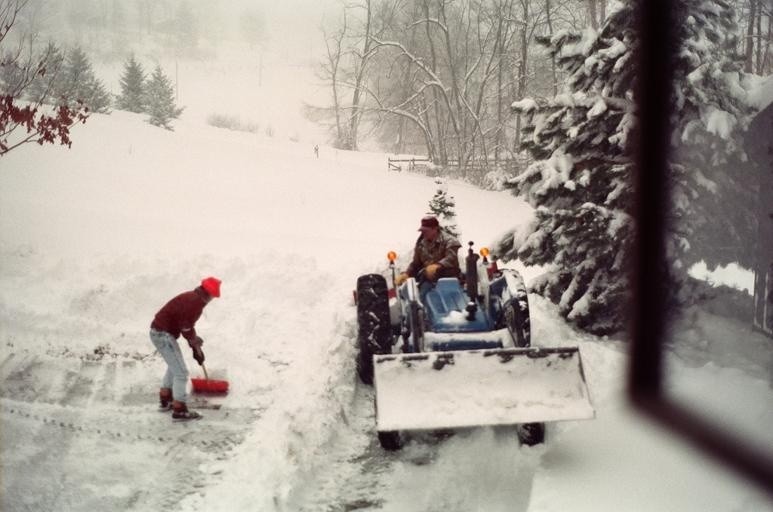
[
  {"xmin": 394, "ymin": 215, "xmax": 464, "ymax": 303},
  {"xmin": 149, "ymin": 276, "xmax": 222, "ymax": 418}
]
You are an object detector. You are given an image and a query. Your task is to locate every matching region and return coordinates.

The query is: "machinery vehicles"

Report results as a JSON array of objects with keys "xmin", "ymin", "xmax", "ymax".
[{"xmin": 352, "ymin": 237, "xmax": 596, "ymax": 451}]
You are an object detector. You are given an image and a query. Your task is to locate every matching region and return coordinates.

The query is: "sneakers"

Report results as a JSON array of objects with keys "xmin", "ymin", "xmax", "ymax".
[{"xmin": 161, "ymin": 395, "xmax": 201, "ymax": 422}]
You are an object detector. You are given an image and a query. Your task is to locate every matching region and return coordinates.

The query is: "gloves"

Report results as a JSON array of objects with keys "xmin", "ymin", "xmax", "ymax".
[
  {"xmin": 426, "ymin": 263, "xmax": 442, "ymax": 282},
  {"xmin": 189, "ymin": 337, "xmax": 204, "ymax": 366},
  {"xmin": 392, "ymin": 273, "xmax": 409, "ymax": 286}
]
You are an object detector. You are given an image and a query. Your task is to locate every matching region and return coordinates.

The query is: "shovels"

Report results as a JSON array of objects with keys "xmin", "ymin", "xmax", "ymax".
[{"xmin": 191, "ymin": 345, "xmax": 229, "ymax": 393}]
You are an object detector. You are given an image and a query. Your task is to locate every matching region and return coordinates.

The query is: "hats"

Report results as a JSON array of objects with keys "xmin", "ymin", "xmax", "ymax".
[
  {"xmin": 202, "ymin": 278, "xmax": 221, "ymax": 297},
  {"xmin": 418, "ymin": 217, "xmax": 439, "ymax": 231}
]
